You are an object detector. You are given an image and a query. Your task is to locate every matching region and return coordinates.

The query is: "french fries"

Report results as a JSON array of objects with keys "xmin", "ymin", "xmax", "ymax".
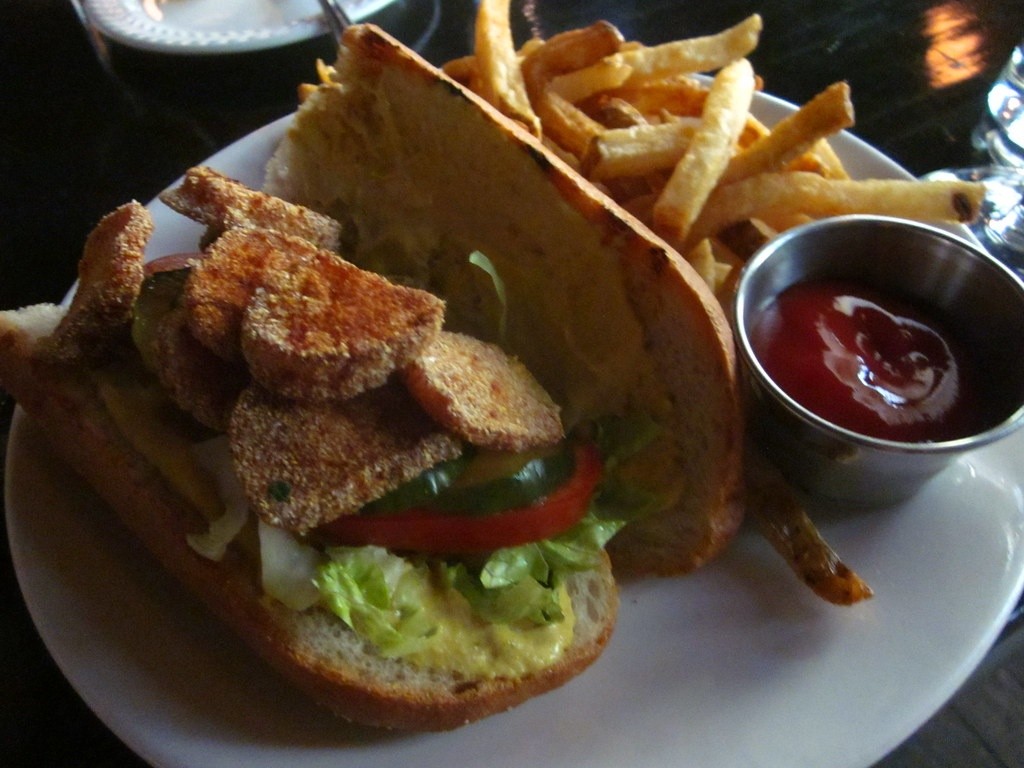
[{"xmin": 296, "ymin": 0, "xmax": 987, "ymax": 600}]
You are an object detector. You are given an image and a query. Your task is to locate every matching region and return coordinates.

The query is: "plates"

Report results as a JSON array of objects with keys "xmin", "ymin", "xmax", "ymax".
[
  {"xmin": 74, "ymin": 0, "xmax": 396, "ymax": 56},
  {"xmin": 4, "ymin": 55, "xmax": 1024, "ymax": 767}
]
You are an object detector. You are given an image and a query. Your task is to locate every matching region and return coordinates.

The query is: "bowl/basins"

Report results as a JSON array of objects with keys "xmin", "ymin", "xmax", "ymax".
[{"xmin": 734, "ymin": 214, "xmax": 1024, "ymax": 515}]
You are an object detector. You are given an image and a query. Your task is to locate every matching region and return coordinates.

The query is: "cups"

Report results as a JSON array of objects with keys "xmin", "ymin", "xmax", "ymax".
[{"xmin": 987, "ymin": 41, "xmax": 1024, "ymax": 168}]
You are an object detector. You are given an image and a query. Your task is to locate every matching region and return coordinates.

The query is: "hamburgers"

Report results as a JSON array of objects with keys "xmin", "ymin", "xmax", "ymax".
[{"xmin": 0, "ymin": 20, "xmax": 750, "ymax": 736}]
